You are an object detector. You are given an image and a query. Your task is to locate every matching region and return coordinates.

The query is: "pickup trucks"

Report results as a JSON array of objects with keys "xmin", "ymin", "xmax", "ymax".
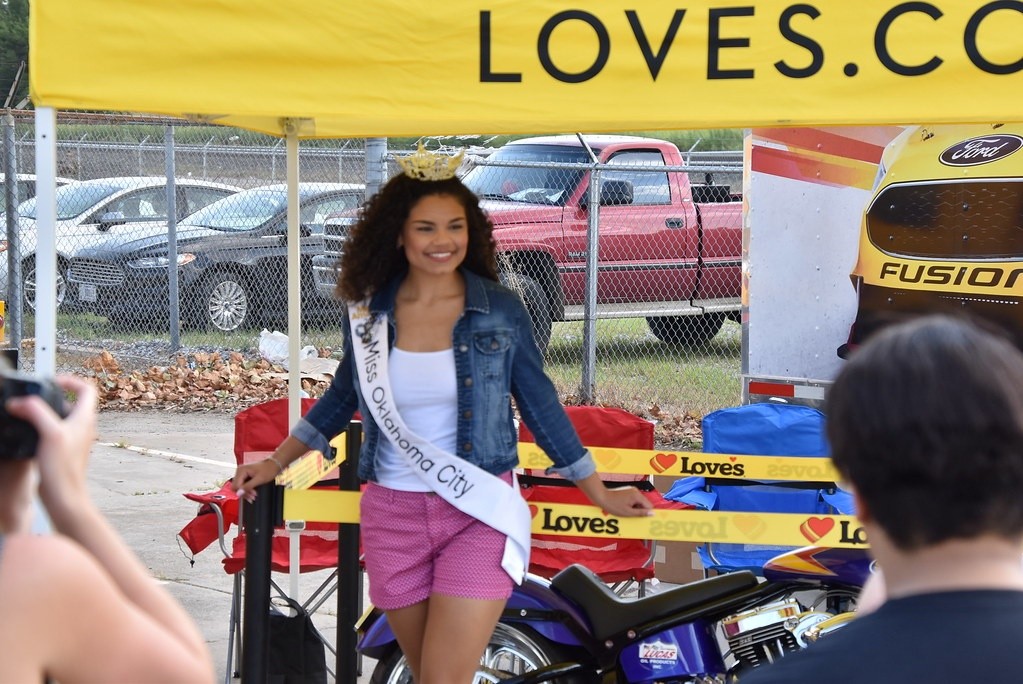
[{"xmin": 311, "ymin": 134, "xmax": 743, "ymax": 359}]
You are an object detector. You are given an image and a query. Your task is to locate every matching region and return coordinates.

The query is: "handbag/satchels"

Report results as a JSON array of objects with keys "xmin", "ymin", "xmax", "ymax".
[{"xmin": 268, "ymin": 595, "xmax": 328, "ymax": 684}]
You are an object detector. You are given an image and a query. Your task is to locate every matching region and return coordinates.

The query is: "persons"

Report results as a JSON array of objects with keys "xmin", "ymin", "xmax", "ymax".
[
  {"xmin": 741, "ymin": 314, "xmax": 1023, "ymax": 684},
  {"xmin": 230, "ymin": 138, "xmax": 654, "ymax": 684},
  {"xmin": 0, "ymin": 374, "xmax": 214, "ymax": 684}
]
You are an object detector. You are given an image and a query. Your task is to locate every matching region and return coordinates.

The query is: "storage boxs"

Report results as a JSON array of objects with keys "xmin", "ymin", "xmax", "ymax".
[{"xmin": 654, "ymin": 475, "xmax": 717, "ymax": 585}]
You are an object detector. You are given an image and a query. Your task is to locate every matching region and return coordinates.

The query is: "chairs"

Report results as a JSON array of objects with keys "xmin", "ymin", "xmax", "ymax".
[
  {"xmin": 664, "ymin": 403, "xmax": 857, "ymax": 578},
  {"xmin": 516, "ymin": 405, "xmax": 656, "ymax": 598},
  {"xmin": 174, "ymin": 397, "xmax": 365, "ymax": 684}
]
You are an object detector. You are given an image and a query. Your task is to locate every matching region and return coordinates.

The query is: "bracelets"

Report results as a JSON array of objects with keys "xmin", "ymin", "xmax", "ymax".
[{"xmin": 267, "ymin": 448, "xmax": 290, "ymax": 475}]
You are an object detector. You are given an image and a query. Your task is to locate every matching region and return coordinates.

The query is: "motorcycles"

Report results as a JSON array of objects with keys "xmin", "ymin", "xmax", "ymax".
[{"xmin": 355, "ymin": 545, "xmax": 876, "ymax": 683}]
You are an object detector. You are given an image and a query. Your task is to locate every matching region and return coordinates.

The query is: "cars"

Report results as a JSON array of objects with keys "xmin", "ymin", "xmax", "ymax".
[
  {"xmin": 61, "ymin": 183, "xmax": 366, "ymax": 336},
  {"xmin": 0, "ymin": 176, "xmax": 279, "ymax": 316}
]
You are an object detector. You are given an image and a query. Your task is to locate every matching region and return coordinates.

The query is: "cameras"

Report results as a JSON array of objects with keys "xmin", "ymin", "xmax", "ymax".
[{"xmin": 0, "ymin": 369, "xmax": 74, "ymax": 463}]
